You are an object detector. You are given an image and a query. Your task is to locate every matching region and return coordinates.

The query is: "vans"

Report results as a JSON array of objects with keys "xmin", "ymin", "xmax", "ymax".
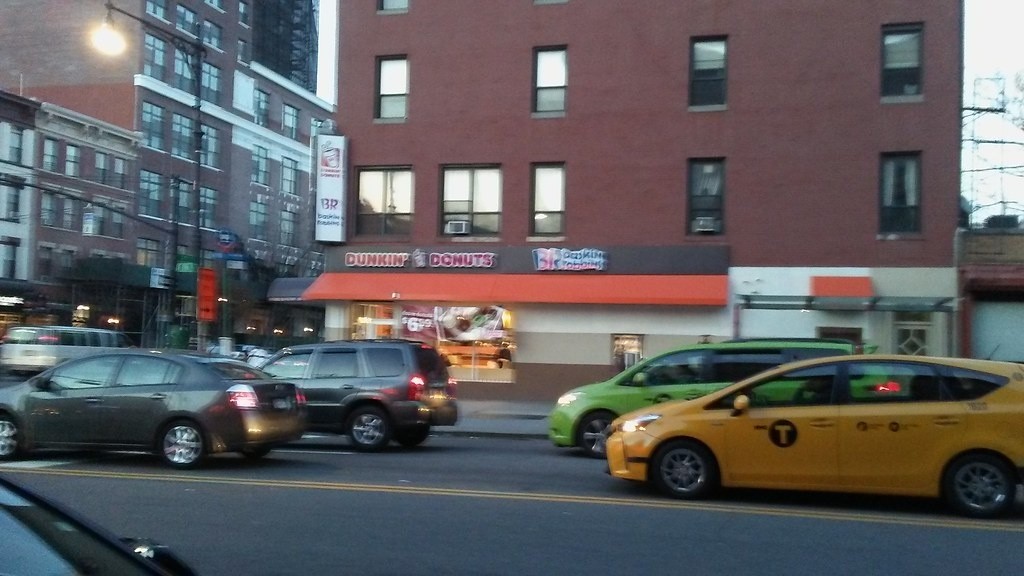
[{"xmin": 4, "ymin": 327, "xmax": 134, "ymax": 381}]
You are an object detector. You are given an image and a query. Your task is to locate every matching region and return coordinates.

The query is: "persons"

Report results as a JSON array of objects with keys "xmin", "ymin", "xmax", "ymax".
[
  {"xmin": 789, "ymin": 367, "xmax": 832, "ymax": 406},
  {"xmin": 244, "ymin": 335, "xmax": 272, "ymax": 373},
  {"xmin": 908, "ymin": 374, "xmax": 937, "ymax": 403},
  {"xmin": 655, "ymin": 356, "xmax": 696, "ymax": 385}
]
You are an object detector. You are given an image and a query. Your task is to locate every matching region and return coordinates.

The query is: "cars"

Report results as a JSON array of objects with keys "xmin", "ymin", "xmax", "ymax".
[
  {"xmin": 601, "ymin": 350, "xmax": 1024, "ymax": 519},
  {"xmin": 260, "ymin": 338, "xmax": 461, "ymax": 455},
  {"xmin": 2, "ymin": 347, "xmax": 309, "ymax": 472},
  {"xmin": 550, "ymin": 341, "xmax": 889, "ymax": 456}
]
